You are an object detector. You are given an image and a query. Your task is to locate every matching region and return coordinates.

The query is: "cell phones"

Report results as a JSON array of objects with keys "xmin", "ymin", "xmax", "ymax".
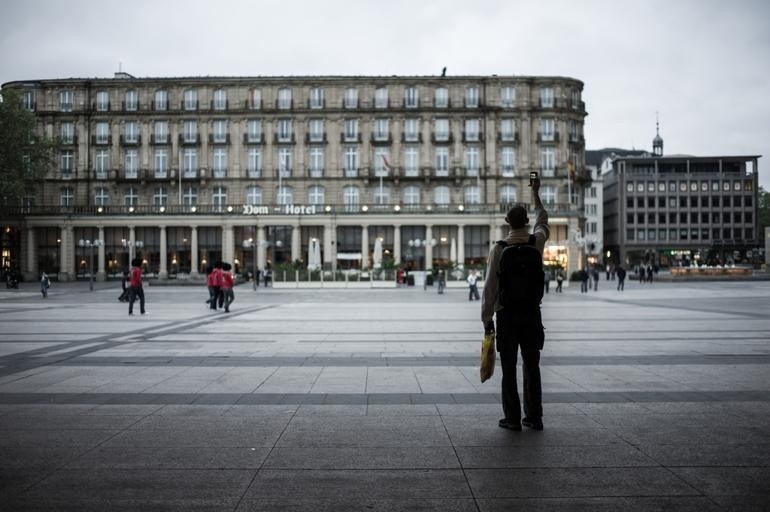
[{"xmin": 530, "ymin": 172, "xmax": 538, "ymax": 184}]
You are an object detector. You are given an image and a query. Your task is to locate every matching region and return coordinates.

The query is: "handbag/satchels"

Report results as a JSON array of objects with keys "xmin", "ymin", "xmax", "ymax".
[{"xmin": 481, "ymin": 333, "xmax": 497, "ymax": 383}]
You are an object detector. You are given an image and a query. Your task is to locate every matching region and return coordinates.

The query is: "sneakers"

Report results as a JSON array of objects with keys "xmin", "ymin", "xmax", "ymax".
[
  {"xmin": 498, "ymin": 417, "xmax": 523, "ymax": 432},
  {"xmin": 521, "ymin": 416, "xmax": 544, "ymax": 431}
]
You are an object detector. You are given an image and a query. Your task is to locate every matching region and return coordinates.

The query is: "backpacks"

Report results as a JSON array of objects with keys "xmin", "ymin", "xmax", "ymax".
[{"xmin": 495, "ymin": 233, "xmax": 545, "ymax": 311}]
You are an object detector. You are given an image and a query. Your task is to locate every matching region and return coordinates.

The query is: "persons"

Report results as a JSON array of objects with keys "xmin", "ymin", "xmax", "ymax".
[
  {"xmin": 205, "ymin": 261, "xmax": 235, "ymax": 313},
  {"xmin": 40, "ymin": 272, "xmax": 51, "ymax": 298},
  {"xmin": 434, "ymin": 268, "xmax": 447, "ymax": 293},
  {"xmin": 256, "ymin": 265, "xmax": 269, "ymax": 287},
  {"xmin": 581, "ymin": 261, "xmax": 658, "ymax": 293},
  {"xmin": 128, "ymin": 258, "xmax": 145, "ymax": 314},
  {"xmin": 556, "ymin": 270, "xmax": 564, "ymax": 293},
  {"xmin": 544, "ymin": 267, "xmax": 551, "ymax": 293},
  {"xmin": 467, "ymin": 268, "xmax": 480, "ymax": 300},
  {"xmin": 3, "ymin": 268, "xmax": 19, "ymax": 289},
  {"xmin": 118, "ymin": 271, "xmax": 129, "ymax": 301},
  {"xmin": 480, "ymin": 173, "xmax": 550, "ymax": 431}
]
